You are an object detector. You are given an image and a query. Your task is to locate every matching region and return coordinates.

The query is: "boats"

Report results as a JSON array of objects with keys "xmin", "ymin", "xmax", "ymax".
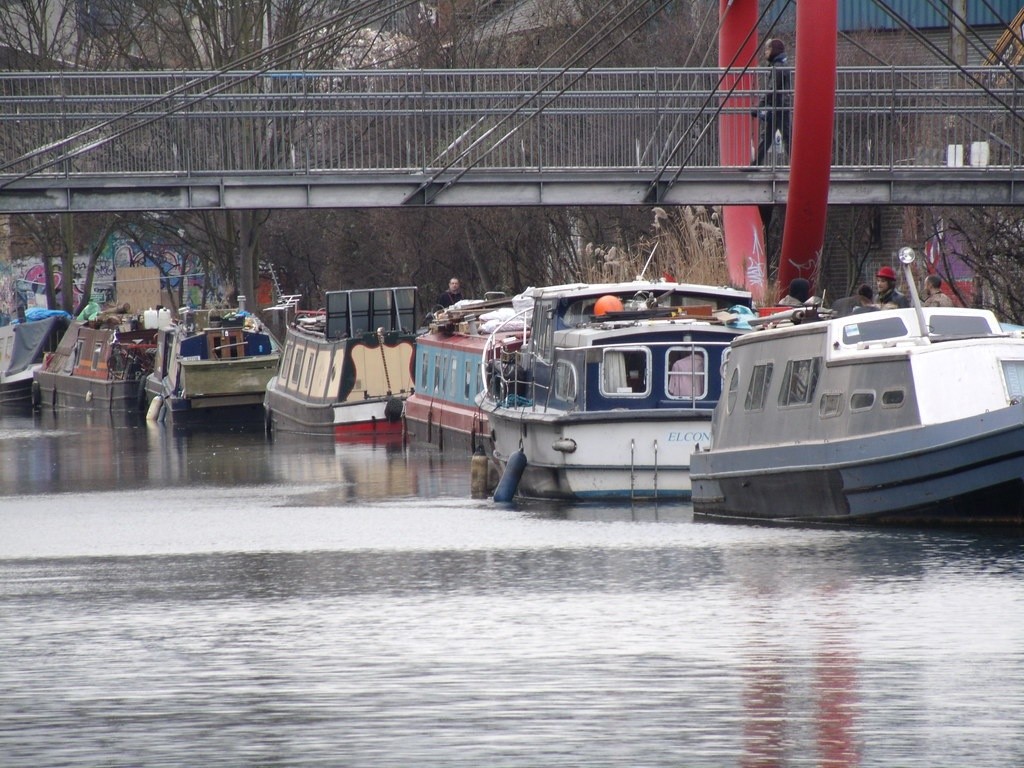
[
  {"xmin": 472, "ymin": 244, "xmax": 761, "ymax": 501},
  {"xmin": 263, "ymin": 286, "xmax": 430, "ymax": 438},
  {"xmin": 404, "ymin": 292, "xmax": 558, "ymax": 455},
  {"xmin": 144, "ymin": 295, "xmax": 283, "ymax": 426},
  {"xmin": 30, "ymin": 303, "xmax": 158, "ymax": 412},
  {"xmin": 689, "ymin": 248, "xmax": 1023, "ymax": 524},
  {"xmin": 0, "ymin": 307, "xmax": 74, "ymax": 398}
]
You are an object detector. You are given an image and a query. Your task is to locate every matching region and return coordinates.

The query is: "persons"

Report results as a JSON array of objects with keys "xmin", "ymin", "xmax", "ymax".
[
  {"xmin": 873, "ymin": 266, "xmax": 909, "ymax": 308},
  {"xmin": 741, "ymin": 39, "xmax": 792, "ymax": 171},
  {"xmin": 778, "ymin": 279, "xmax": 811, "ymax": 309},
  {"xmin": 854, "ymin": 284, "xmax": 880, "ymax": 313},
  {"xmin": 420, "ymin": 304, "xmax": 443, "ymax": 329},
  {"xmin": 923, "ymin": 274, "xmax": 955, "ymax": 308},
  {"xmin": 437, "ymin": 277, "xmax": 463, "ymax": 308}
]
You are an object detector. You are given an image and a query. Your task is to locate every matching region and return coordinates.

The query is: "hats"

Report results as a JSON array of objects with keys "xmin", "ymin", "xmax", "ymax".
[
  {"xmin": 876, "ymin": 266, "xmax": 896, "ymax": 282},
  {"xmin": 789, "ymin": 279, "xmax": 809, "ymax": 302}
]
